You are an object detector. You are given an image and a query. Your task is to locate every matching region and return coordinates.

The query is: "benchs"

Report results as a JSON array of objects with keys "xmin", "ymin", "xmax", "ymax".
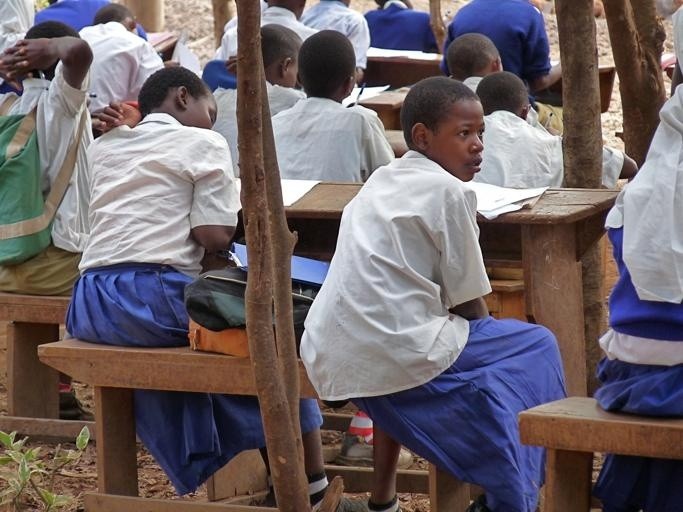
[{"xmin": 36, "ymin": 339, "xmax": 470, "ymax": 512}]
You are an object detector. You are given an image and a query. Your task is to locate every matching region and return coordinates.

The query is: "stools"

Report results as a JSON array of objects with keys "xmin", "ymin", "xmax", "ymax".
[
  {"xmin": 0, "ymin": 293, "xmax": 98, "ymax": 447},
  {"xmin": 517, "ymin": 395, "xmax": 683, "ymax": 512}
]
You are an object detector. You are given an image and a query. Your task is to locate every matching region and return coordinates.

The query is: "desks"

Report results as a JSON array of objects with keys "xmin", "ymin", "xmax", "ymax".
[
  {"xmin": 234, "ymin": 179, "xmax": 623, "ymax": 397},
  {"xmin": 145, "ymin": 28, "xmax": 178, "ymax": 62},
  {"xmin": 366, "ymin": 45, "xmax": 616, "ymax": 116},
  {"xmin": 348, "ymin": 83, "xmax": 409, "ymax": 133}
]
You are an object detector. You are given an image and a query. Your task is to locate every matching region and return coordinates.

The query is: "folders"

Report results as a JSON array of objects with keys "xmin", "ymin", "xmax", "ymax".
[{"xmin": 231, "ymin": 242, "xmax": 330, "ymax": 286}]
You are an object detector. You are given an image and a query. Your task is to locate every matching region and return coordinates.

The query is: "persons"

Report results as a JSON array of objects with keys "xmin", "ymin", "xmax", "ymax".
[
  {"xmin": 467, "ymin": 72, "xmax": 638, "ymax": 191},
  {"xmin": 364, "ymin": 0, "xmax": 439, "ymax": 54},
  {"xmin": 76, "ymin": 3, "xmax": 163, "ymax": 136},
  {"xmin": 442, "ymin": 0, "xmax": 562, "ymax": 133},
  {"xmin": 212, "ymin": 24, "xmax": 303, "ymax": 181},
  {"xmin": 35, "ymin": 0, "xmax": 148, "ymax": 45},
  {"xmin": 269, "ymin": 29, "xmax": 412, "ymax": 472},
  {"xmin": 1, "ymin": 21, "xmax": 95, "ymax": 263},
  {"xmin": 296, "ymin": 77, "xmax": 567, "ymax": 512},
  {"xmin": 71, "ymin": 66, "xmax": 328, "ymax": 503},
  {"xmin": 299, "ymin": 0, "xmax": 371, "ymax": 86},
  {"xmin": 595, "ymin": 4, "xmax": 683, "ymax": 507},
  {"xmin": 218, "ymin": 0, "xmax": 319, "ymax": 70}
]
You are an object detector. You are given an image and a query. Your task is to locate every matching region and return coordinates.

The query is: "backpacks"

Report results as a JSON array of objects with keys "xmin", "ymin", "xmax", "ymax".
[{"xmin": 0, "ymin": 95, "xmax": 87, "ymax": 268}]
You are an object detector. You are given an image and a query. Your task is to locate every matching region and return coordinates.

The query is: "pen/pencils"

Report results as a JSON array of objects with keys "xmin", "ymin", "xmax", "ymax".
[
  {"xmin": 46, "ymin": 87, "xmax": 97, "ymax": 99},
  {"xmin": 354, "ymin": 82, "xmax": 367, "ymax": 106},
  {"xmin": 544, "ymin": 112, "xmax": 553, "ymax": 129}
]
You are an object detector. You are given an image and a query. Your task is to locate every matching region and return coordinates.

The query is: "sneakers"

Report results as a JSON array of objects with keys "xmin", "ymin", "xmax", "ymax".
[
  {"xmin": 58, "ymin": 393, "xmax": 97, "ymax": 421},
  {"xmin": 337, "ymin": 433, "xmax": 412, "ymax": 470},
  {"xmin": 338, "ymin": 498, "xmax": 370, "ymax": 512}
]
